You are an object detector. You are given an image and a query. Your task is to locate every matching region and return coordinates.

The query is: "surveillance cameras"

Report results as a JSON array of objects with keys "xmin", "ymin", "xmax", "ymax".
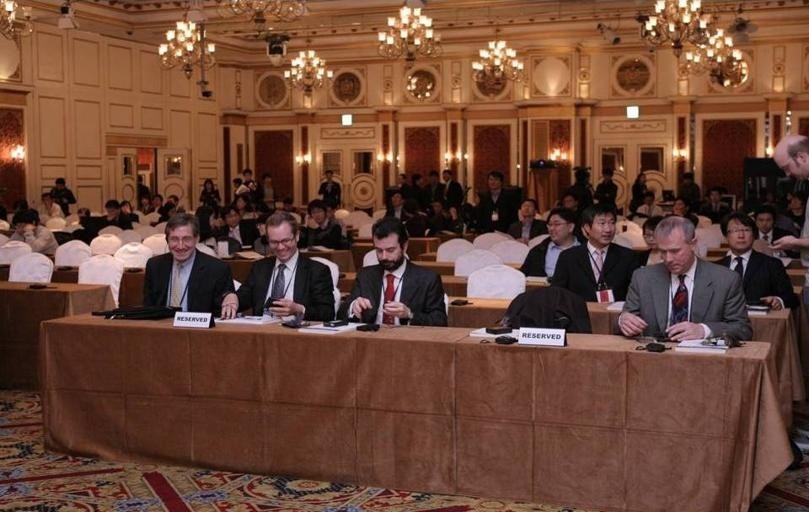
[{"xmin": 598, "ymin": 23, "xmax": 620, "ymax": 45}]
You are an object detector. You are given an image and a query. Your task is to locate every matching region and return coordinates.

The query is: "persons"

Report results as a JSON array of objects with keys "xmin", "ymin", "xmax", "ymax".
[
  {"xmin": 473, "ymin": 169, "xmax": 795, "ymax": 341},
  {"xmin": 772, "ymin": 135, "xmax": 809, "ymax": 450},
  {"xmin": 10, "ymin": 175, "xmax": 180, "ymax": 262},
  {"xmin": 149, "ymin": 170, "xmax": 476, "ymax": 330}
]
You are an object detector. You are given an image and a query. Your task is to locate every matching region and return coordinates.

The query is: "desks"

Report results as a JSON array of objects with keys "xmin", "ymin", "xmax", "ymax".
[
  {"xmin": 39, "ymin": 309, "xmax": 796, "ymax": 512},
  {"xmin": 1, "ymin": 205, "xmax": 804, "ymax": 403}
]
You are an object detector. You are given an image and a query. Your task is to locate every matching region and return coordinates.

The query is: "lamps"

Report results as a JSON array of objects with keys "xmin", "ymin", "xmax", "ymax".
[
  {"xmin": 285, "ymin": 31, "xmax": 336, "ymax": 96},
  {"xmin": 683, "ymin": 24, "xmax": 742, "ymax": 76},
  {"xmin": 214, "ymin": 0, "xmax": 306, "ymax": 23},
  {"xmin": 467, "ymin": 25, "xmax": 530, "ymax": 86},
  {"xmin": 1, "ymin": 0, "xmax": 36, "ymax": 39},
  {"xmin": 371, "ymin": 1, "xmax": 445, "ymax": 65},
  {"xmin": 635, "ymin": 1, "xmax": 710, "ymax": 56},
  {"xmin": 155, "ymin": 0, "xmax": 217, "ymax": 78}
]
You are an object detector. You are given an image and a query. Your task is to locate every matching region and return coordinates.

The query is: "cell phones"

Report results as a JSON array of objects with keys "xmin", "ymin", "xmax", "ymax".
[
  {"xmin": 653, "ymin": 337, "xmax": 681, "ymax": 344},
  {"xmin": 486, "ymin": 326, "xmax": 512, "ymax": 334},
  {"xmin": 323, "ymin": 319, "xmax": 348, "ymax": 326},
  {"xmin": 263, "ymin": 298, "xmax": 284, "ymax": 308}
]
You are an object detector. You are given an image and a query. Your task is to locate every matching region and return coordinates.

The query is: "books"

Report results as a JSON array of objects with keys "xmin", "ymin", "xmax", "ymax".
[{"xmin": 675, "ymin": 339, "xmax": 729, "ymax": 354}]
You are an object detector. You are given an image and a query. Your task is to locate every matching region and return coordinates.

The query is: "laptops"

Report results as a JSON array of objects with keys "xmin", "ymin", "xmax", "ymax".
[{"xmin": 662, "ymin": 189, "xmax": 677, "ymax": 201}]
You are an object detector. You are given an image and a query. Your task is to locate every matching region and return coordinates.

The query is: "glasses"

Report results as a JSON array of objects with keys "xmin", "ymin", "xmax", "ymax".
[{"xmin": 269, "ymin": 236, "xmax": 295, "ymax": 249}]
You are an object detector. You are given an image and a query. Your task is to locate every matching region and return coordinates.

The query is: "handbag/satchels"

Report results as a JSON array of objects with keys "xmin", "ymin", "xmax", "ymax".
[{"xmin": 105, "ymin": 305, "xmax": 173, "ymax": 319}]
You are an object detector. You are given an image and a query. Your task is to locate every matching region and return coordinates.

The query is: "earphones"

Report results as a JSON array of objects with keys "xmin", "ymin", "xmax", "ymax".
[{"xmin": 166, "ymin": 237, "xmax": 169, "ymax": 244}]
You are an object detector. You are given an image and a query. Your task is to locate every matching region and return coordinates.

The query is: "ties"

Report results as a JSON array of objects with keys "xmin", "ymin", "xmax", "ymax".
[
  {"xmin": 763, "ymin": 235, "xmax": 768, "ymax": 241},
  {"xmin": 735, "ymin": 257, "xmax": 743, "ymax": 277},
  {"xmin": 272, "ymin": 264, "xmax": 286, "ymax": 299},
  {"xmin": 170, "ymin": 263, "xmax": 181, "ymax": 308},
  {"xmin": 670, "ymin": 275, "xmax": 688, "ymax": 337},
  {"xmin": 594, "ymin": 249, "xmax": 603, "ymax": 283},
  {"xmin": 383, "ymin": 274, "xmax": 394, "ymax": 324}
]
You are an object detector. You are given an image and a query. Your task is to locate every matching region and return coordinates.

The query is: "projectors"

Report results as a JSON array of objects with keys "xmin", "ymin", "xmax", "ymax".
[{"xmin": 530, "ymin": 158, "xmax": 555, "ymax": 168}]
